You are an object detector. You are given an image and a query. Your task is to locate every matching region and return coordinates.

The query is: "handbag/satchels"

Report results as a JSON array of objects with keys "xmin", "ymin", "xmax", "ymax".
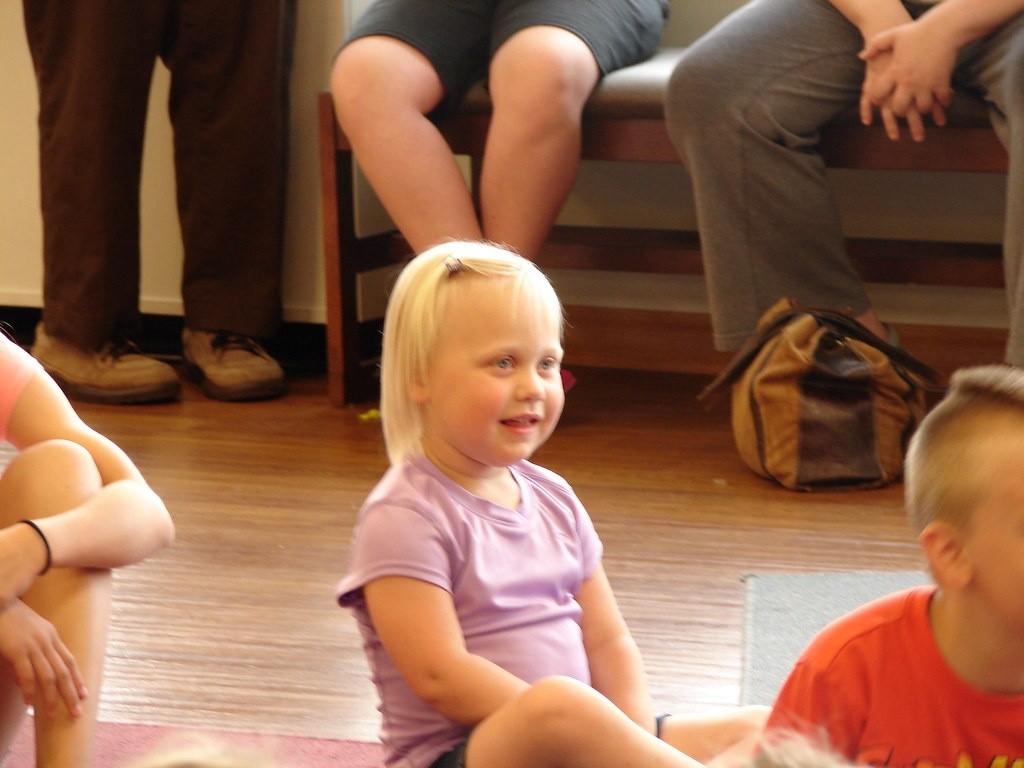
[{"xmin": 697, "ymin": 297, "xmax": 950, "ymax": 493}]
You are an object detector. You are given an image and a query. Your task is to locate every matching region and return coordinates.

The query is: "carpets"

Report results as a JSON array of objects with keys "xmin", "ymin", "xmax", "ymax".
[{"xmin": 741, "ymin": 566, "xmax": 936, "ymax": 705}]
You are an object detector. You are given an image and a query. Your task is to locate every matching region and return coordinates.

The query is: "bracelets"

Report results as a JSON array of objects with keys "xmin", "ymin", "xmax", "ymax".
[{"xmin": 20, "ymin": 519, "xmax": 52, "ymax": 577}]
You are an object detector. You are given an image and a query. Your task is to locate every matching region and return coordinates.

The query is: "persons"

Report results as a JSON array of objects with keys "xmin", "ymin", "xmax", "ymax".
[
  {"xmin": 318, "ymin": 0, "xmax": 669, "ymax": 263},
  {"xmin": 743, "ymin": 364, "xmax": 1023, "ymax": 768},
  {"xmin": 336, "ymin": 240, "xmax": 772, "ymax": 768},
  {"xmin": 24, "ymin": 0, "xmax": 298, "ymax": 405},
  {"xmin": 664, "ymin": 0, "xmax": 1024, "ymax": 368},
  {"xmin": 0, "ymin": 330, "xmax": 174, "ymax": 768}
]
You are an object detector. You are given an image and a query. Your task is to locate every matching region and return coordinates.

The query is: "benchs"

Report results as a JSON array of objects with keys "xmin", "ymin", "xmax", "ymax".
[{"xmin": 316, "ymin": 42, "xmax": 1024, "ymax": 409}]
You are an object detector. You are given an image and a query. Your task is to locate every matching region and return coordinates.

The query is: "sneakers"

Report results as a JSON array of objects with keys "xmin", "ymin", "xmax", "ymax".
[
  {"xmin": 182, "ymin": 328, "xmax": 285, "ymax": 400},
  {"xmin": 27, "ymin": 321, "xmax": 179, "ymax": 403}
]
